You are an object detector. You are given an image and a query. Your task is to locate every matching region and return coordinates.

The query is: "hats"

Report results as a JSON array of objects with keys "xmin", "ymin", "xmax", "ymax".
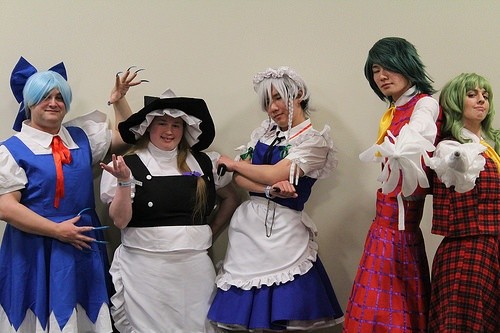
[{"xmin": 117, "ymin": 89, "xmax": 215, "ymax": 151}]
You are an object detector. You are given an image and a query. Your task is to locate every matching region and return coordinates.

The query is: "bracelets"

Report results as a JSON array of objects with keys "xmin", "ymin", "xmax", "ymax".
[
  {"xmin": 264, "ymin": 186, "xmax": 276, "ymax": 199},
  {"xmin": 116, "ymin": 178, "xmax": 142, "ymax": 187}
]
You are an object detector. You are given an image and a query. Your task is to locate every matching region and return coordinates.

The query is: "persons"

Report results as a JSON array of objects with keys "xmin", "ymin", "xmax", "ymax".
[
  {"xmin": 0, "ymin": 57, "xmax": 149, "ymax": 333},
  {"xmin": 99, "ymin": 89, "xmax": 241, "ymax": 333},
  {"xmin": 206, "ymin": 68, "xmax": 343, "ymax": 333},
  {"xmin": 429, "ymin": 73, "xmax": 500, "ymax": 333},
  {"xmin": 344, "ymin": 36, "xmax": 441, "ymax": 333}
]
